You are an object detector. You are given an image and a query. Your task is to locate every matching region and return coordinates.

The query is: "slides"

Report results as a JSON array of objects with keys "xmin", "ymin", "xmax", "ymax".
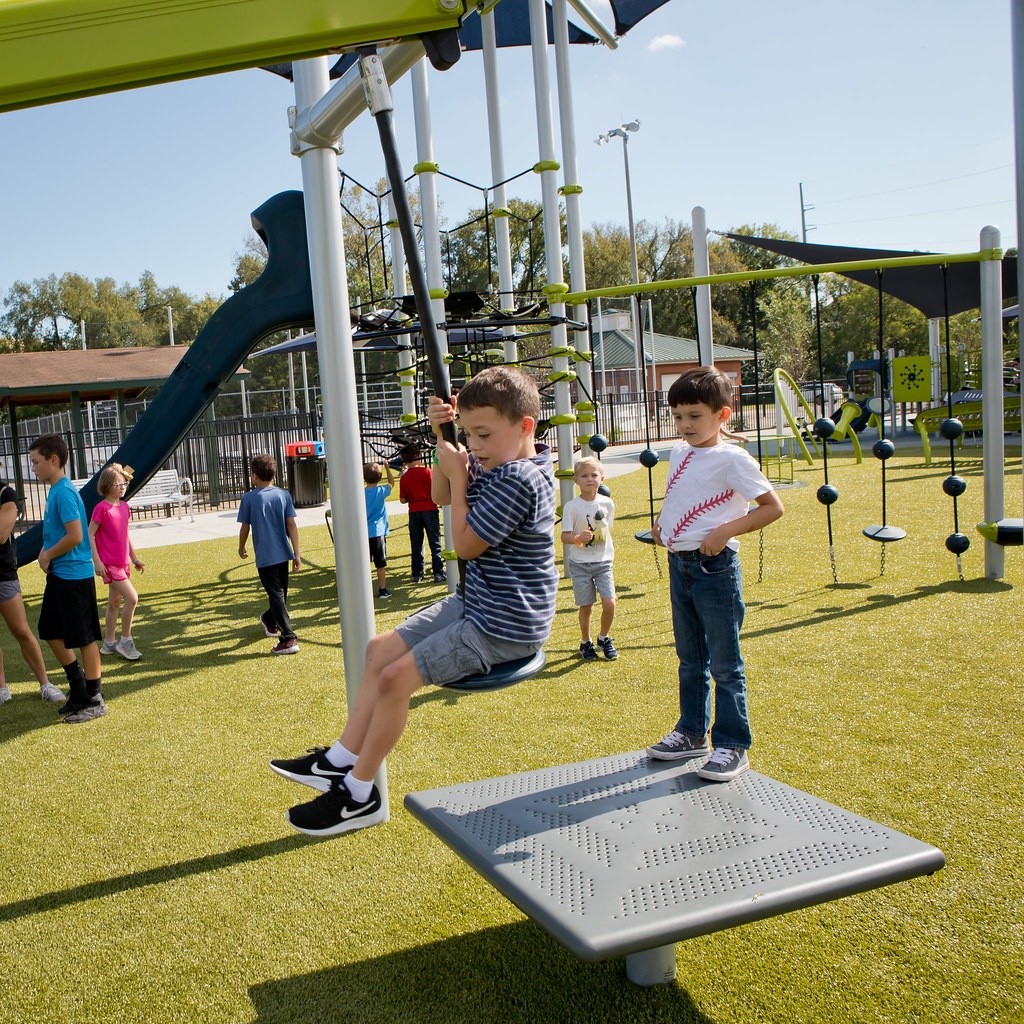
[
  {"xmin": 800, "ymin": 398, "xmax": 863, "ymax": 441},
  {"xmin": 13, "ymin": 189, "xmax": 316, "ymax": 571},
  {"xmin": 823, "ymin": 397, "xmax": 872, "ymax": 442}
]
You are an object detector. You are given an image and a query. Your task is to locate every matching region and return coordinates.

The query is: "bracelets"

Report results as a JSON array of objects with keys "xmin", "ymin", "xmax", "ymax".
[
  {"xmin": 431, "ymin": 448, "xmax": 439, "ymax": 465},
  {"xmin": 134, "ymin": 560, "xmax": 140, "ymax": 565},
  {"xmin": 575, "ymin": 535, "xmax": 583, "ymax": 548}
]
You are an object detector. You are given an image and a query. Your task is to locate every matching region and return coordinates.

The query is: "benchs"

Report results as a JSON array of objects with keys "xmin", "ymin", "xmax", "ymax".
[{"xmin": 70, "ymin": 469, "xmax": 195, "ymax": 523}]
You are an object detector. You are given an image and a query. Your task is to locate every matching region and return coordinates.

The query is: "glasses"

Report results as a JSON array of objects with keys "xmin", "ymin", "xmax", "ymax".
[{"xmin": 113, "ymin": 482, "xmax": 128, "ymax": 488}]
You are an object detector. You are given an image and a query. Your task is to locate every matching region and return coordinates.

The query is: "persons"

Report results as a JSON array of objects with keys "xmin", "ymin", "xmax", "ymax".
[
  {"xmin": 363, "ymin": 459, "xmax": 395, "ymax": 598},
  {"xmin": 645, "ymin": 365, "xmax": 784, "ymax": 781},
  {"xmin": 388, "ymin": 442, "xmax": 408, "ymax": 477},
  {"xmin": 29, "ymin": 433, "xmax": 107, "ymax": 724},
  {"xmin": 719, "ymin": 422, "xmax": 749, "ymax": 443},
  {"xmin": 1006, "ymin": 358, "xmax": 1021, "ymax": 393},
  {"xmin": 236, "ymin": 454, "xmax": 301, "ymax": 654},
  {"xmin": 561, "ymin": 456, "xmax": 619, "ymax": 661},
  {"xmin": 88, "ymin": 466, "xmax": 146, "ymax": 660},
  {"xmin": 0, "ymin": 483, "xmax": 65, "ymax": 704},
  {"xmin": 270, "ymin": 366, "xmax": 557, "ymax": 837},
  {"xmin": 399, "ymin": 442, "xmax": 447, "ymax": 582}
]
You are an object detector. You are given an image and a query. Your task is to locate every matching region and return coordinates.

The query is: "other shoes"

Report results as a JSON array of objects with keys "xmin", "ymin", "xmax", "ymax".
[
  {"xmin": 434, "ymin": 570, "xmax": 447, "ymax": 581},
  {"xmin": 411, "ymin": 576, "xmax": 423, "ymax": 582},
  {"xmin": 0, "ymin": 686, "xmax": 12, "ymax": 703},
  {"xmin": 58, "ymin": 677, "xmax": 107, "ymax": 724},
  {"xmin": 379, "ymin": 588, "xmax": 392, "ymax": 598},
  {"xmin": 270, "ymin": 635, "xmax": 300, "ymax": 654},
  {"xmin": 40, "ymin": 682, "xmax": 66, "ymax": 703},
  {"xmin": 260, "ymin": 612, "xmax": 278, "ymax": 637}
]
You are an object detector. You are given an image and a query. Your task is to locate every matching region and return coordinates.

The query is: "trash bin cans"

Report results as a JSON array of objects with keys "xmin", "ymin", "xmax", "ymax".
[{"xmin": 284, "ymin": 441, "xmax": 328, "ymax": 508}]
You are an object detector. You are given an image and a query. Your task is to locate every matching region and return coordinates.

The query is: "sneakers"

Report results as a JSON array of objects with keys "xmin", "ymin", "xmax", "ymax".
[
  {"xmin": 269, "ymin": 746, "xmax": 354, "ymax": 793},
  {"xmin": 287, "ymin": 779, "xmax": 385, "ymax": 837},
  {"xmin": 100, "ymin": 640, "xmax": 120, "ymax": 654},
  {"xmin": 115, "ymin": 640, "xmax": 143, "ymax": 660},
  {"xmin": 597, "ymin": 635, "xmax": 617, "ymax": 660},
  {"xmin": 579, "ymin": 641, "xmax": 599, "ymax": 660},
  {"xmin": 646, "ymin": 730, "xmax": 709, "ymax": 761},
  {"xmin": 698, "ymin": 747, "xmax": 749, "ymax": 782}
]
